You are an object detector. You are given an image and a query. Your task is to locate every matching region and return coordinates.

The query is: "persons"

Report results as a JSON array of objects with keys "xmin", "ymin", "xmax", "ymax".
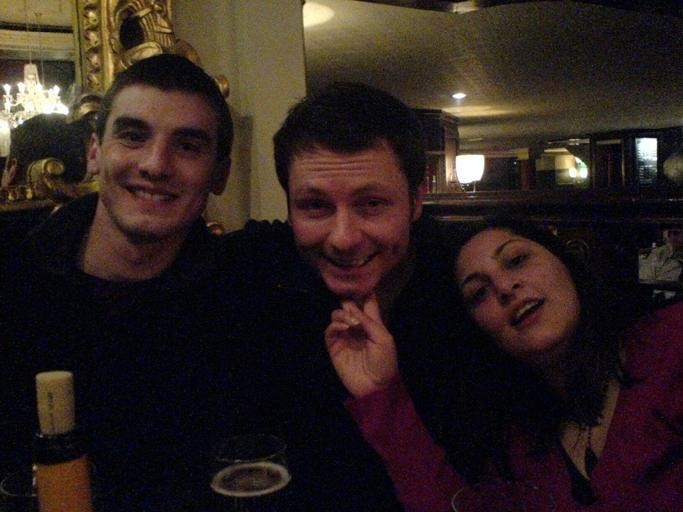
[
  {"xmin": 648, "ymin": 224, "xmax": 682, "ymax": 283},
  {"xmin": 231, "ymin": 85, "xmax": 510, "ymax": 511},
  {"xmin": 0, "ymin": 53, "xmax": 232, "ymax": 511},
  {"xmin": 323, "ymin": 218, "xmax": 681, "ymax": 509},
  {"xmin": 0, "ymin": 114, "xmax": 88, "ymax": 203}
]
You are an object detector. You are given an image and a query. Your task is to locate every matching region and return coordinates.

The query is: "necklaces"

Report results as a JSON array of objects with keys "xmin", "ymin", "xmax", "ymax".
[{"xmin": 573, "ymin": 413, "xmax": 601, "ymax": 476}]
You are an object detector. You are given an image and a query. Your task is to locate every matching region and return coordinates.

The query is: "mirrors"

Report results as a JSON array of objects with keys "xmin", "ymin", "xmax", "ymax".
[{"xmin": 0, "ymin": 1, "xmax": 111, "ymax": 212}]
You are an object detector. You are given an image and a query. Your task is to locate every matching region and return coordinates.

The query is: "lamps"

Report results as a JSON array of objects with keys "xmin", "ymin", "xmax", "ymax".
[{"xmin": 1, "ymin": 1, "xmax": 72, "ymax": 159}]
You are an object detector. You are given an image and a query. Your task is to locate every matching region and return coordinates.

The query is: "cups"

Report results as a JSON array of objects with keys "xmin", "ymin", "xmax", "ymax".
[
  {"xmin": 35, "ymin": 371, "xmax": 79, "ymax": 435},
  {"xmin": 209, "ymin": 435, "xmax": 289, "ymax": 511},
  {"xmin": 26, "ymin": 441, "xmax": 93, "ymax": 510}
]
